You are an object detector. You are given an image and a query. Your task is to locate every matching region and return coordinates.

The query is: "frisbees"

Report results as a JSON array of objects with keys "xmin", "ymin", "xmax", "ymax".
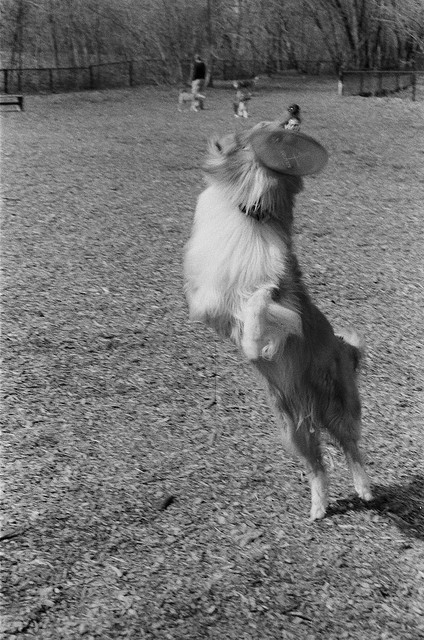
[{"xmin": 250, "ymin": 130, "xmax": 328, "ymax": 176}]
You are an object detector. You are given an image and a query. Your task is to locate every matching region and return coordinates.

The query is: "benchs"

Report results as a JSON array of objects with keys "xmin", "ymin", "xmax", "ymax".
[{"xmin": 0, "ymin": 93, "xmax": 25, "ymax": 111}]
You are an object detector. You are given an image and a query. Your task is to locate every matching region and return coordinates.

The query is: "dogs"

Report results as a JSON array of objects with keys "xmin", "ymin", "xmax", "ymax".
[{"xmin": 179, "ymin": 105, "xmax": 376, "ymax": 520}]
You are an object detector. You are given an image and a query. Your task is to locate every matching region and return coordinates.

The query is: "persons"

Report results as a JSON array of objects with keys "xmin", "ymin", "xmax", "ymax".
[
  {"xmin": 190, "ymin": 53, "xmax": 206, "ymax": 112},
  {"xmin": 231, "ymin": 79, "xmax": 253, "ymax": 120}
]
